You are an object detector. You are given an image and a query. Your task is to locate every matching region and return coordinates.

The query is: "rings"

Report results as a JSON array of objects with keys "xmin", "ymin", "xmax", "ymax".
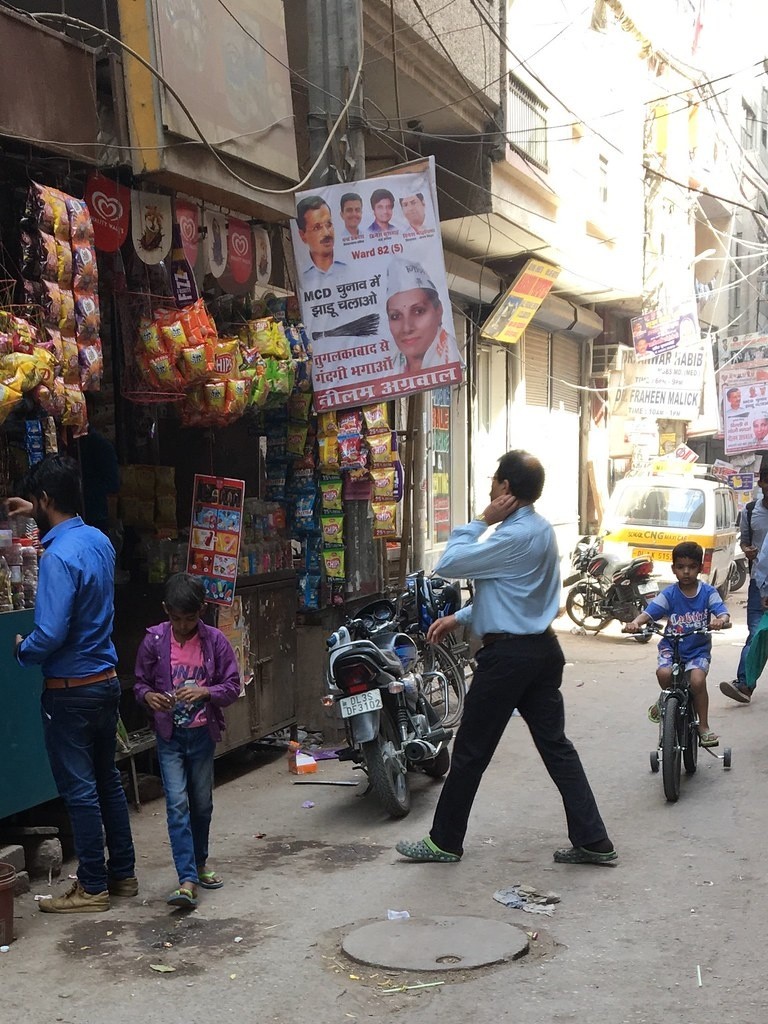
[{"xmin": 500, "ymin": 499, "xmax": 504, "ymax": 503}]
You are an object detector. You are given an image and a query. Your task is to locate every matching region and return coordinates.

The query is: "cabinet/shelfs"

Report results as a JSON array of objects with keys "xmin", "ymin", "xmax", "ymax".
[{"xmin": 116, "ymin": 568, "xmax": 302, "ymax": 775}]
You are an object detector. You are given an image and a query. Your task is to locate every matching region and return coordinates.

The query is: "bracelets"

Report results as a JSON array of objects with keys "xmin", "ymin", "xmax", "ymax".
[{"xmin": 476, "ymin": 514, "xmax": 486, "ymax": 521}]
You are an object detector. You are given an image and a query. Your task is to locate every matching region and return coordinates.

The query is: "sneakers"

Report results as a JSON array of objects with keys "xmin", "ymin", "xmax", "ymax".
[
  {"xmin": 38, "ymin": 880, "xmax": 109, "ymax": 912},
  {"xmin": 719, "ymin": 679, "xmax": 751, "ymax": 703},
  {"xmin": 106, "ymin": 876, "xmax": 138, "ymax": 896}
]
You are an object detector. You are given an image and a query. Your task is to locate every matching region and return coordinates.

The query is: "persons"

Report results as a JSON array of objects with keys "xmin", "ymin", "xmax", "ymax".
[
  {"xmin": 394, "ymin": 449, "xmax": 620, "ymax": 868},
  {"xmin": 632, "ymin": 315, "xmax": 696, "ymax": 361},
  {"xmin": 5, "ymin": 451, "xmax": 140, "ymax": 915},
  {"xmin": 718, "ymin": 452, "xmax": 767, "ymax": 703},
  {"xmin": 293, "ymin": 185, "xmax": 459, "ymax": 383},
  {"xmin": 132, "ymin": 570, "xmax": 241, "ymax": 911},
  {"xmin": 724, "ymin": 383, "xmax": 768, "ymax": 445},
  {"xmin": 624, "ymin": 542, "xmax": 731, "ymax": 748}
]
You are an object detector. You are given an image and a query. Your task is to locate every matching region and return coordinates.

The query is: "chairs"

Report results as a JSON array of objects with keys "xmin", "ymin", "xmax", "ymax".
[{"xmin": 639, "ymin": 491, "xmax": 668, "ymax": 520}]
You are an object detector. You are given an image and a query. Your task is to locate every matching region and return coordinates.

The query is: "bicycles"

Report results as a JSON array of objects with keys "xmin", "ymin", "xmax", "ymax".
[
  {"xmin": 413, "ymin": 567, "xmax": 480, "ymax": 731},
  {"xmin": 621, "ymin": 619, "xmax": 733, "ymax": 804}
]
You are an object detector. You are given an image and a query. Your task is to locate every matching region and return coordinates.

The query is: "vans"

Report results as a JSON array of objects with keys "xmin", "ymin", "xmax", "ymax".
[{"xmin": 597, "ymin": 475, "xmax": 748, "ymax": 602}]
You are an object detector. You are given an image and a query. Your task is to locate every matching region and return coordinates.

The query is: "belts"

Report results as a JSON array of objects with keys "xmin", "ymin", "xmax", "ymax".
[
  {"xmin": 484, "ymin": 626, "xmax": 554, "ymax": 646},
  {"xmin": 42, "ymin": 666, "xmax": 116, "ymax": 689}
]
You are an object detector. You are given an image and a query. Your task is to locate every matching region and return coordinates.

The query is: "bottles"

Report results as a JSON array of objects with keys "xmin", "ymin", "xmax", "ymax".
[
  {"xmin": 148, "ymin": 533, "xmax": 172, "ymax": 584},
  {"xmin": 0, "ymin": 537, "xmax": 45, "ymax": 611}
]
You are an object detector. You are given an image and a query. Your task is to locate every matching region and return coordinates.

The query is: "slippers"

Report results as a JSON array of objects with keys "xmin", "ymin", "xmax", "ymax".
[
  {"xmin": 395, "ymin": 838, "xmax": 460, "ymax": 862},
  {"xmin": 701, "ymin": 730, "xmax": 719, "ymax": 746},
  {"xmin": 198, "ymin": 866, "xmax": 223, "ymax": 889},
  {"xmin": 166, "ymin": 888, "xmax": 198, "ymax": 906},
  {"xmin": 649, "ymin": 702, "xmax": 662, "ymax": 723},
  {"xmin": 553, "ymin": 844, "xmax": 617, "ymax": 864}
]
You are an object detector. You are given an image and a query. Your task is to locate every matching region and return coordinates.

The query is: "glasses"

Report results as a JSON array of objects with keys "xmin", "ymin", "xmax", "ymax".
[{"xmin": 487, "ymin": 475, "xmax": 499, "ymax": 484}]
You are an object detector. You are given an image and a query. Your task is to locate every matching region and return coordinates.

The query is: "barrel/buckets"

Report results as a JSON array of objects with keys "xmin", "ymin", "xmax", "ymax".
[{"xmin": 0, "ymin": 863, "xmax": 16, "ymax": 947}]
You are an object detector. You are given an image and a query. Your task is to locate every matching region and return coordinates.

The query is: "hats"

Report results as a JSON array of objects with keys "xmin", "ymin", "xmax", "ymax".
[{"xmin": 385, "ymin": 257, "xmax": 438, "ymax": 305}]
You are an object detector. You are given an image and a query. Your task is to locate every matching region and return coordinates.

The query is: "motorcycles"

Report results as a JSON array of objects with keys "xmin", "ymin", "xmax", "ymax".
[
  {"xmin": 563, "ymin": 527, "xmax": 663, "ymax": 645},
  {"xmin": 321, "ymin": 586, "xmax": 452, "ymax": 820}
]
